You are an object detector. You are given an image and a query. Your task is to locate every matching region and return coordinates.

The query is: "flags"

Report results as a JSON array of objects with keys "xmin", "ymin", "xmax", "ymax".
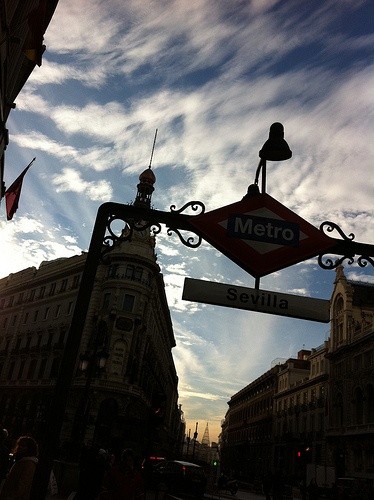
[{"xmin": 5, "ymin": 171, "xmax": 26, "ymax": 220}]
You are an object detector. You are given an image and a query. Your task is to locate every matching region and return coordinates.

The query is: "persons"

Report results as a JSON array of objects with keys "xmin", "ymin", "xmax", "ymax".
[
  {"xmin": 0, "ymin": 435, "xmax": 38, "ymax": 500},
  {"xmin": 261, "ymin": 468, "xmax": 285, "ymax": 500},
  {"xmin": 307, "ymin": 482, "xmax": 321, "ymax": 500},
  {"xmin": 254, "ymin": 472, "xmax": 262, "ymax": 494},
  {"xmin": 0, "ymin": 429, "xmax": 9, "ymax": 483},
  {"xmin": 105, "ymin": 449, "xmax": 145, "ymax": 500},
  {"xmin": 302, "ymin": 480, "xmax": 307, "ymax": 500},
  {"xmin": 79, "ymin": 439, "xmax": 104, "ymax": 500},
  {"xmin": 144, "ymin": 456, "xmax": 161, "ymax": 500}
]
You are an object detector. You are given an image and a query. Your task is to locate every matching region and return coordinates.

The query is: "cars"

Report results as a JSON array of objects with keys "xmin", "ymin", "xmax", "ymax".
[{"xmin": 140, "ymin": 456, "xmax": 208, "ymax": 495}]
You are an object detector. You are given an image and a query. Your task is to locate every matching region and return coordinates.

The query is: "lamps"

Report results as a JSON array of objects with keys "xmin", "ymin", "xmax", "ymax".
[{"xmin": 255, "ymin": 122, "xmax": 293, "ymax": 185}]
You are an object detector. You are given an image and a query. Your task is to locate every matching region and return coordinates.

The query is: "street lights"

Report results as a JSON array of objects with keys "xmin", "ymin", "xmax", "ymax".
[{"xmin": 77, "ymin": 337, "xmax": 110, "ymax": 420}]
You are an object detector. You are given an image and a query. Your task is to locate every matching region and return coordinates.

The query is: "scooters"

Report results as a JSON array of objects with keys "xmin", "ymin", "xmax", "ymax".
[{"xmin": 214, "ymin": 471, "xmax": 240, "ymax": 494}]
